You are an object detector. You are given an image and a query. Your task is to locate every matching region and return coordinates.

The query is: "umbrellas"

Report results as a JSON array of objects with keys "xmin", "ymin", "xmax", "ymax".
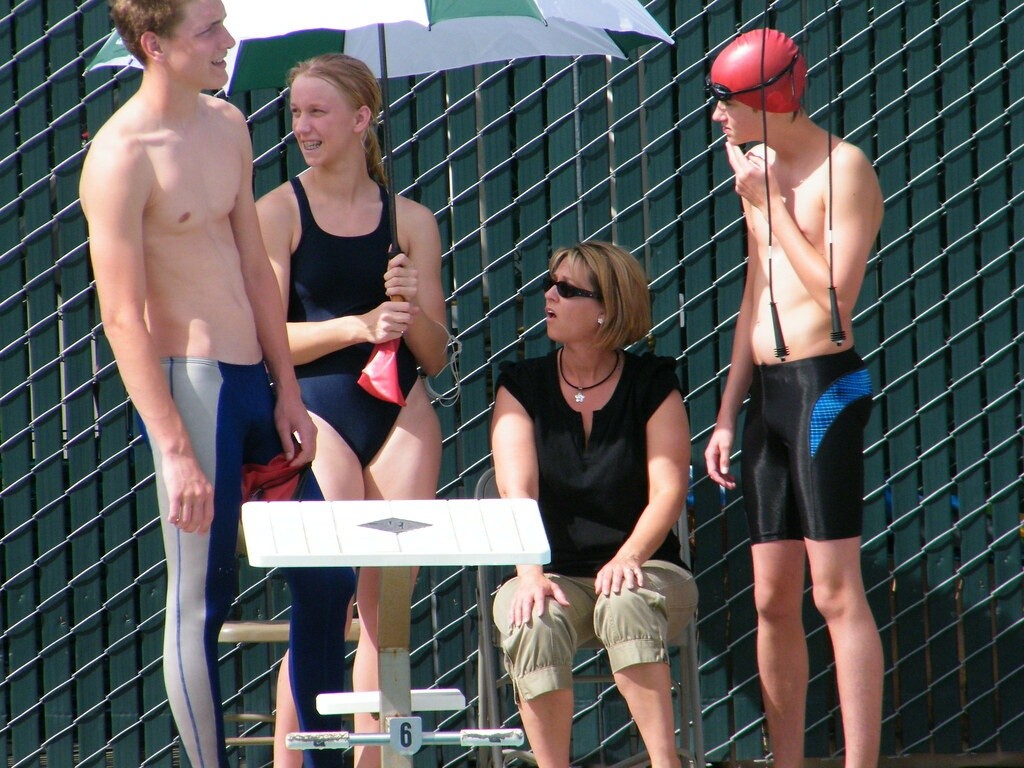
[{"xmin": 80, "ymin": 1, "xmax": 678, "ymax": 303}]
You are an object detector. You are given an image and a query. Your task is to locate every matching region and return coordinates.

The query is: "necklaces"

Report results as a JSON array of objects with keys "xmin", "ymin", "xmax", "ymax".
[{"xmin": 559, "ymin": 343, "xmax": 620, "ymax": 403}]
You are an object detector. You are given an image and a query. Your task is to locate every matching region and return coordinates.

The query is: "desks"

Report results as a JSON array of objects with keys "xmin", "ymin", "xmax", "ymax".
[{"xmin": 241, "ymin": 498, "xmax": 553, "ymax": 768}]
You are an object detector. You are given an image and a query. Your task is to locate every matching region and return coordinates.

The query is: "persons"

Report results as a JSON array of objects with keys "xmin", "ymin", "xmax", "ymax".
[
  {"xmin": 249, "ymin": 51, "xmax": 452, "ymax": 768},
  {"xmin": 702, "ymin": 26, "xmax": 887, "ymax": 768},
  {"xmin": 488, "ymin": 239, "xmax": 699, "ymax": 768},
  {"xmin": 79, "ymin": 0, "xmax": 361, "ymax": 768}
]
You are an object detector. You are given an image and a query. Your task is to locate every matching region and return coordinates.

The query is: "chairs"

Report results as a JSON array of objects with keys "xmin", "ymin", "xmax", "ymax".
[{"xmin": 474, "ymin": 468, "xmax": 708, "ymax": 768}]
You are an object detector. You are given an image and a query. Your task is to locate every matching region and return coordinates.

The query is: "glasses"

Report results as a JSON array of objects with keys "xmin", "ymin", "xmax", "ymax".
[
  {"xmin": 704, "ymin": 49, "xmax": 801, "ymax": 101},
  {"xmin": 543, "ymin": 277, "xmax": 603, "ymax": 300}
]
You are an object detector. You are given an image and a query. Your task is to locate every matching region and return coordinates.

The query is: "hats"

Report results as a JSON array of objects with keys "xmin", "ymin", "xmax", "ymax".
[
  {"xmin": 356, "ymin": 337, "xmax": 407, "ymax": 407},
  {"xmin": 711, "ymin": 28, "xmax": 806, "ymax": 113},
  {"xmin": 239, "ymin": 444, "xmax": 302, "ymax": 526}
]
